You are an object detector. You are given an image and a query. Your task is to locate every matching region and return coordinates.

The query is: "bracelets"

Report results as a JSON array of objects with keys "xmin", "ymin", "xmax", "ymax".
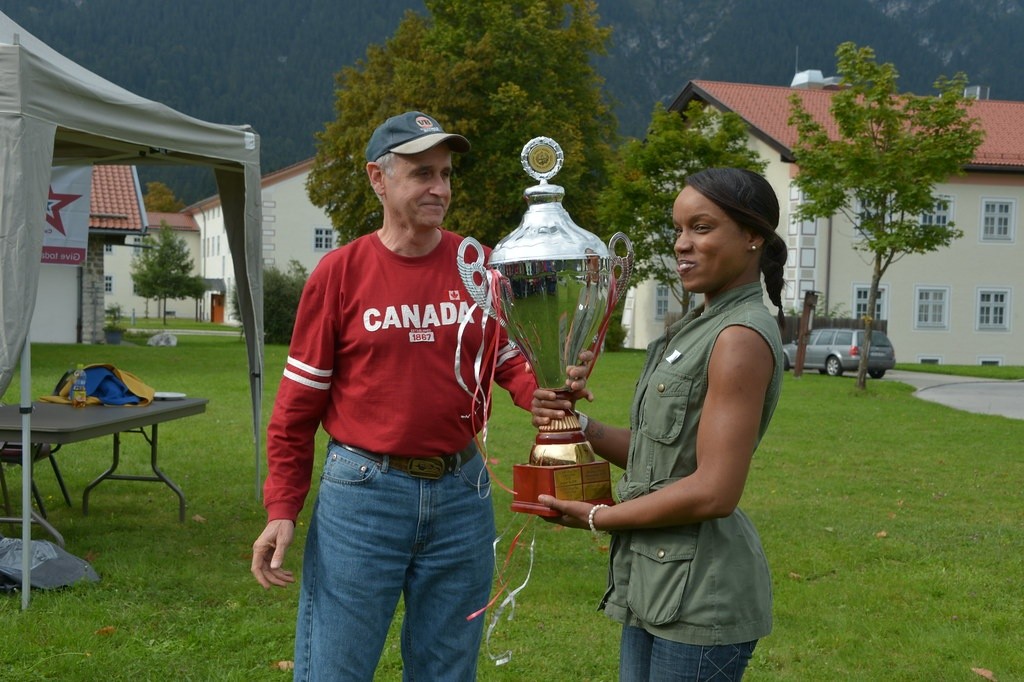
[{"xmin": 588, "ymin": 503, "xmax": 611, "ymax": 539}]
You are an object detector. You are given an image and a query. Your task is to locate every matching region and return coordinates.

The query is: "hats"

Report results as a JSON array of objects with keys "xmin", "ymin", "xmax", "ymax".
[{"xmin": 365, "ymin": 111, "xmax": 471, "ymax": 163}]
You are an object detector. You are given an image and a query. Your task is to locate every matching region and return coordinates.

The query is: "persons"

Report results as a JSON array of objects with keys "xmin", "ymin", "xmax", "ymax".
[
  {"xmin": 535, "ymin": 168, "xmax": 788, "ymax": 681},
  {"xmin": 250, "ymin": 111, "xmax": 593, "ymax": 682}
]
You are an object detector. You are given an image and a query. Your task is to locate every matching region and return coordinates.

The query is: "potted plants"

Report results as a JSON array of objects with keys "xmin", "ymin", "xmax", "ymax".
[{"xmin": 102, "ymin": 304, "xmax": 127, "ymax": 345}]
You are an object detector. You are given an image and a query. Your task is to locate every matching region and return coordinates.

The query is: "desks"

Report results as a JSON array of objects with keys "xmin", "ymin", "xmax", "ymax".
[{"xmin": 0, "ymin": 397, "xmax": 210, "ymax": 547}]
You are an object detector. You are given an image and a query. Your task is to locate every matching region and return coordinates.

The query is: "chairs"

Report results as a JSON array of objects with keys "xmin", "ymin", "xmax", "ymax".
[{"xmin": 0, "ymin": 405, "xmax": 73, "ymax": 531}]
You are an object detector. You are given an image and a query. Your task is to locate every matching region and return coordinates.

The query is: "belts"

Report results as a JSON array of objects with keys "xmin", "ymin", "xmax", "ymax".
[{"xmin": 331, "ymin": 437, "xmax": 478, "ymax": 480}]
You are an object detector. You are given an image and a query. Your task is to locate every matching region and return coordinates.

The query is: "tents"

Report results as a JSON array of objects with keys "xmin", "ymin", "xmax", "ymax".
[{"xmin": 0, "ymin": 7, "xmax": 265, "ymax": 610}]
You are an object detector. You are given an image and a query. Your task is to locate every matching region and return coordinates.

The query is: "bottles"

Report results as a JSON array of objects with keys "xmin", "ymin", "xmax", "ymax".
[{"xmin": 72, "ymin": 363, "xmax": 86, "ymax": 408}]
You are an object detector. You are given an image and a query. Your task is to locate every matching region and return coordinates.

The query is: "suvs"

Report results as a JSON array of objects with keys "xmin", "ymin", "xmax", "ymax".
[{"xmin": 782, "ymin": 327, "xmax": 896, "ymax": 379}]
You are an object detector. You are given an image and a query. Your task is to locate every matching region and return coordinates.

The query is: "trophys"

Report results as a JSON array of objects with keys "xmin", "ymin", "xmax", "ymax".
[{"xmin": 457, "ymin": 136, "xmax": 632, "ymax": 515}]
